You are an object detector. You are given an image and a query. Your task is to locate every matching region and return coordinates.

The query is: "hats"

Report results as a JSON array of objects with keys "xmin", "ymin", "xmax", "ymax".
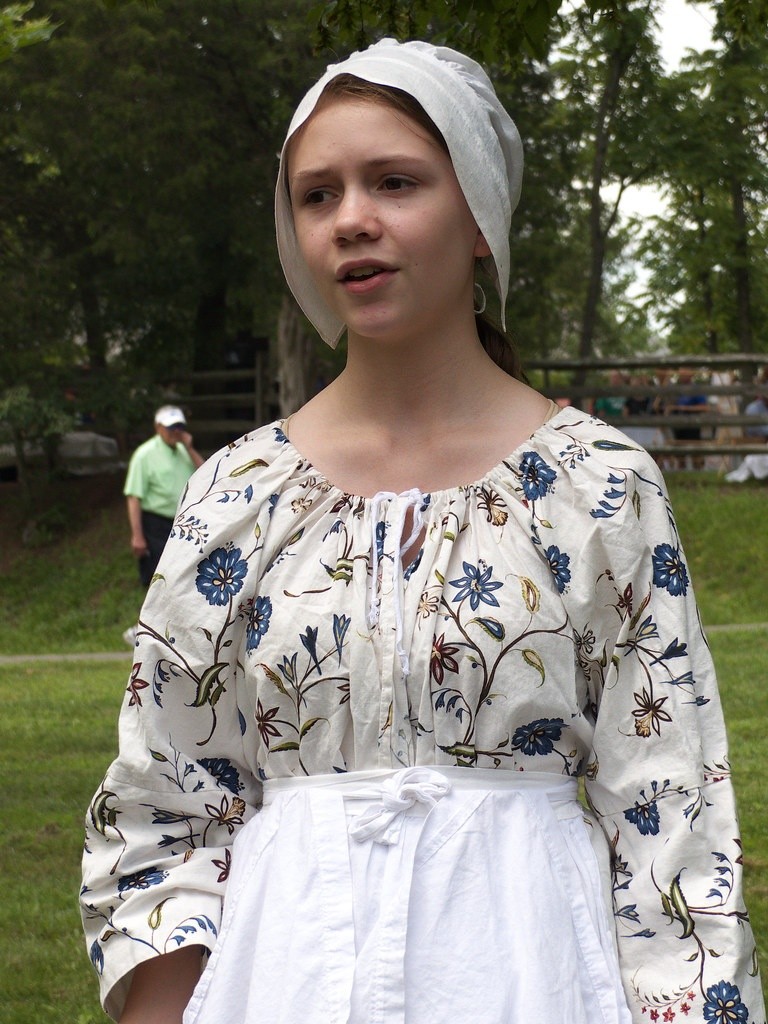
[
  {"xmin": 155, "ymin": 404, "xmax": 190, "ymax": 433},
  {"xmin": 274, "ymin": 38, "xmax": 525, "ymax": 349}
]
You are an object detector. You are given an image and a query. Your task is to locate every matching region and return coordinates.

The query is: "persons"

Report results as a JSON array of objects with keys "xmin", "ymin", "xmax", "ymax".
[
  {"xmin": 82, "ymin": 37, "xmax": 765, "ymax": 1024},
  {"xmin": 124, "ymin": 404, "xmax": 206, "ymax": 596},
  {"xmin": 610, "ymin": 364, "xmax": 767, "ymax": 481}
]
